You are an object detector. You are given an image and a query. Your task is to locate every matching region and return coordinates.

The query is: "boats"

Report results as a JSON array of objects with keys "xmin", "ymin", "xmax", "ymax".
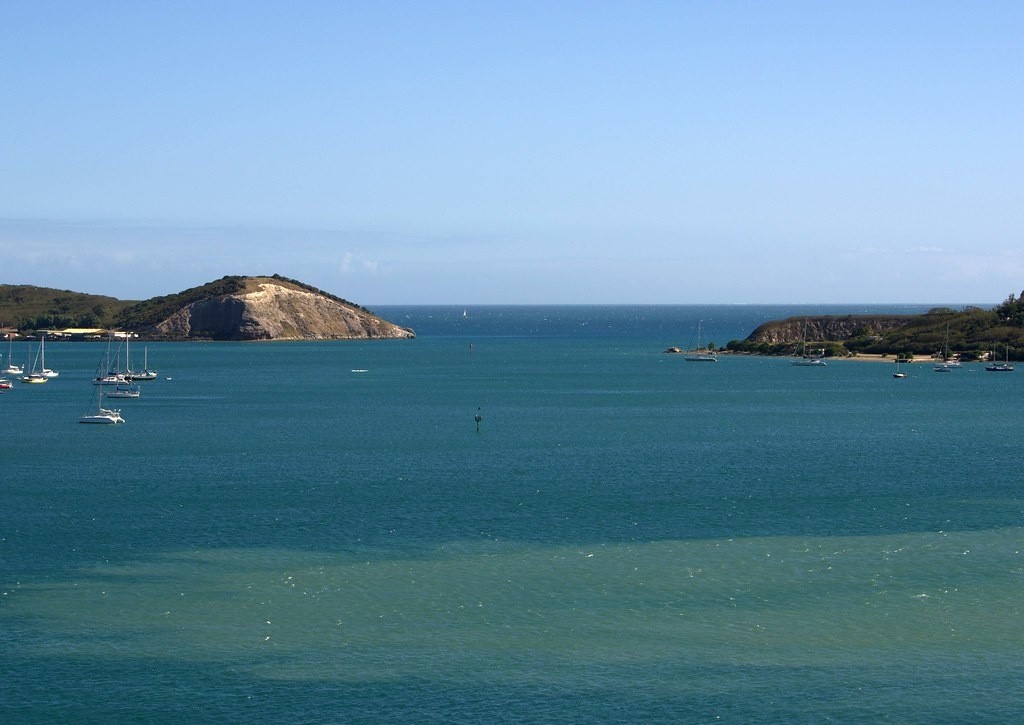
[
  {"xmin": 0, "ymin": 378, "xmax": 13, "ymax": 394},
  {"xmin": 934, "ymin": 367, "xmax": 952, "ymax": 372}
]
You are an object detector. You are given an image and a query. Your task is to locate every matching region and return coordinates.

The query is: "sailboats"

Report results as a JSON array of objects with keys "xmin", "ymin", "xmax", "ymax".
[
  {"xmin": 107, "ymin": 331, "xmax": 131, "ymax": 376},
  {"xmin": 30, "ymin": 336, "xmax": 59, "ymax": 377},
  {"xmin": 20, "ymin": 344, "xmax": 48, "ymax": 383},
  {"xmin": 790, "ymin": 319, "xmax": 828, "ymax": 366},
  {"xmin": 92, "ymin": 327, "xmax": 129, "ymax": 385},
  {"xmin": 1, "ymin": 336, "xmax": 23, "ymax": 374},
  {"xmin": 986, "ymin": 343, "xmax": 1014, "ymax": 371},
  {"xmin": 106, "ymin": 347, "xmax": 141, "ymax": 398},
  {"xmin": 935, "ymin": 325, "xmax": 962, "ymax": 368},
  {"xmin": 79, "ymin": 361, "xmax": 126, "ymax": 424},
  {"xmin": 685, "ymin": 323, "xmax": 718, "ymax": 362},
  {"xmin": 124, "ymin": 345, "xmax": 158, "ymax": 379},
  {"xmin": 893, "ymin": 355, "xmax": 907, "ymax": 378}
]
